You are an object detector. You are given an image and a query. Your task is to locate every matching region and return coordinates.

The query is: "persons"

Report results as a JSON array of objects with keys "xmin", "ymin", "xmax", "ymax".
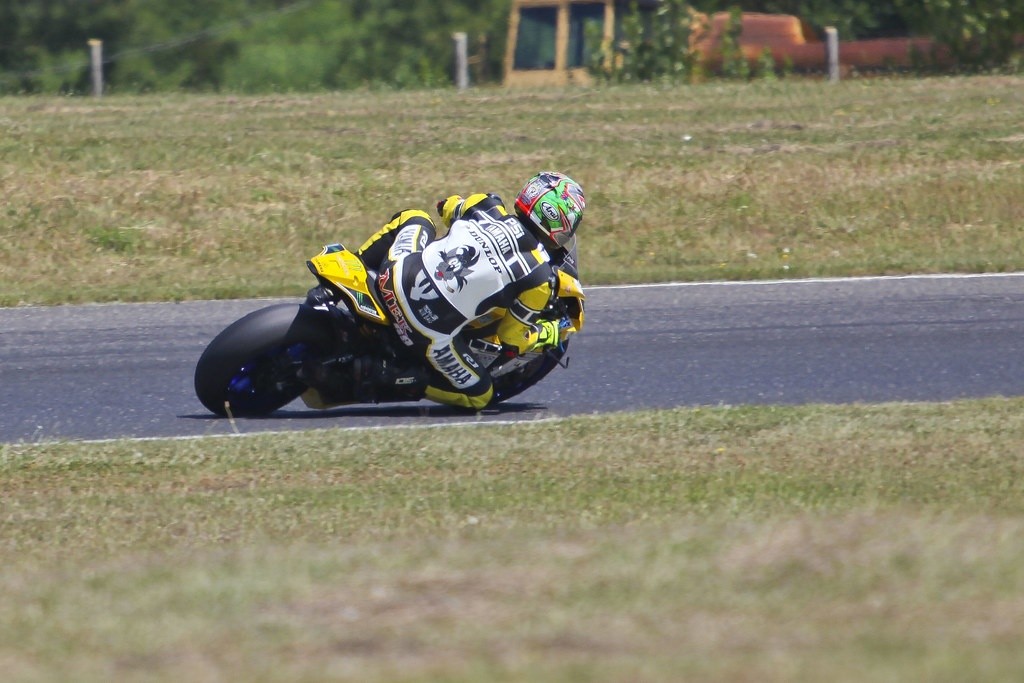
[{"xmin": 352, "ymin": 170, "xmax": 586, "ymax": 409}]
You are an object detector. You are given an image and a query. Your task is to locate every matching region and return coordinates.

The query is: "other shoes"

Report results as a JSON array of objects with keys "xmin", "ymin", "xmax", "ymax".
[
  {"xmin": 307, "ymin": 281, "xmax": 341, "ymax": 304},
  {"xmin": 395, "ymin": 371, "xmax": 428, "ymax": 401}
]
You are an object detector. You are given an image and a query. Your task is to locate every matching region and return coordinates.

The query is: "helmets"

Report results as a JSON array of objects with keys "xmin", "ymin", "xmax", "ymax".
[{"xmin": 514, "ymin": 172, "xmax": 585, "ymax": 251}]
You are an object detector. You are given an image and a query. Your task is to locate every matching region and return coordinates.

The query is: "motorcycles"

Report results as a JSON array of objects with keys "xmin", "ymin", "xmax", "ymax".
[{"xmin": 191, "ymin": 231, "xmax": 586, "ymax": 419}]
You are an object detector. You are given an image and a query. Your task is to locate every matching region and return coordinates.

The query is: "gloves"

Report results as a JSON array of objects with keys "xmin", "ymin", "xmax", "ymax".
[{"xmin": 437, "ymin": 198, "xmax": 448, "ymax": 217}]
